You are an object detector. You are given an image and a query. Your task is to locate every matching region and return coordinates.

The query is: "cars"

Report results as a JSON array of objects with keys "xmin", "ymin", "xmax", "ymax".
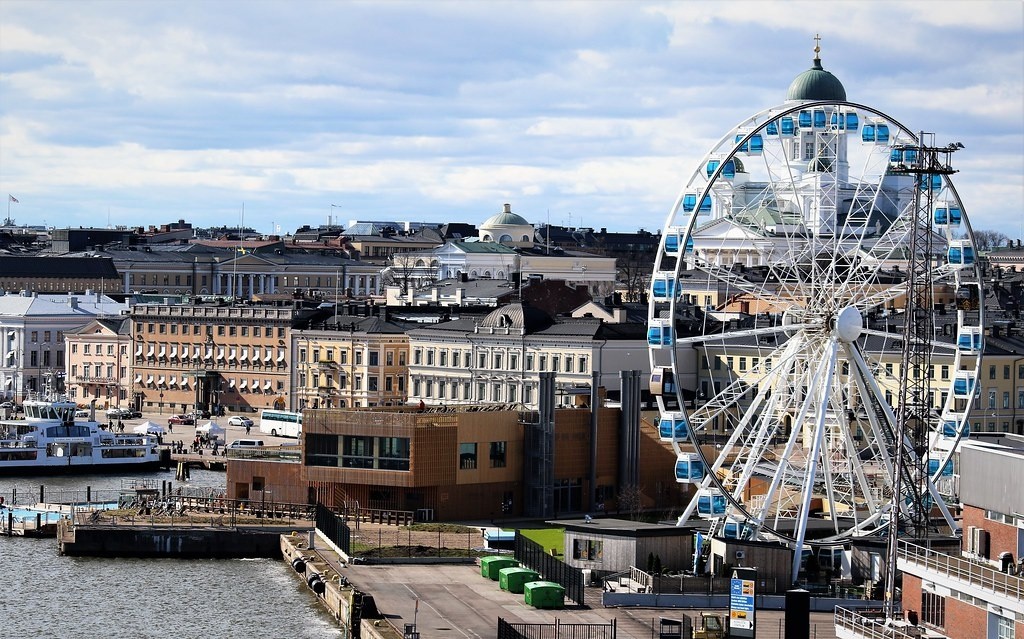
[
  {"xmin": 187, "ymin": 410, "xmax": 211, "ymax": 419},
  {"xmin": 168, "ymin": 414, "xmax": 194, "ymax": 425},
  {"xmin": 65, "ymin": 408, "xmax": 88, "ymax": 417},
  {"xmin": 105, "ymin": 409, "xmax": 132, "ymax": 420},
  {"xmin": 228, "ymin": 416, "xmax": 254, "ymax": 427},
  {"xmin": 0, "ymin": 401, "xmax": 23, "ymax": 413},
  {"xmin": 120, "ymin": 408, "xmax": 142, "ymax": 419}
]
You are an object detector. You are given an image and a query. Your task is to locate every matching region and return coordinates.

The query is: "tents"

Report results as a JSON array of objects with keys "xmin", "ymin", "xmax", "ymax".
[
  {"xmin": 133, "ymin": 421, "xmax": 164, "ymax": 443},
  {"xmin": 195, "ymin": 421, "xmax": 226, "ymax": 444}
]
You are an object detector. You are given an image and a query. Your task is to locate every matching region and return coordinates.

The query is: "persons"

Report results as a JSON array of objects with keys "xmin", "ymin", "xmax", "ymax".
[
  {"xmin": 168, "ymin": 422, "xmax": 173, "ymax": 433},
  {"xmin": 213, "ymin": 442, "xmax": 218, "ymax": 451},
  {"xmin": 191, "ymin": 432, "xmax": 210, "ymax": 452},
  {"xmin": 171, "ymin": 440, "xmax": 183, "ymax": 450},
  {"xmin": 109, "ymin": 419, "xmax": 114, "ymax": 432},
  {"xmin": 116, "ymin": 420, "xmax": 125, "ymax": 432},
  {"xmin": 148, "ymin": 432, "xmax": 162, "ymax": 445},
  {"xmin": 246, "ymin": 425, "xmax": 250, "ymax": 435}
]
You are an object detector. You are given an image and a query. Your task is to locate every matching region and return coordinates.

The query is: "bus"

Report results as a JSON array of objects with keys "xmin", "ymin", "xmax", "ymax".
[{"xmin": 260, "ymin": 410, "xmax": 302, "ymax": 439}]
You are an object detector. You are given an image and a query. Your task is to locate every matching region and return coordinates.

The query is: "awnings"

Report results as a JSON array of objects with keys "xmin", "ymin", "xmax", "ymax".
[
  {"xmin": 227, "ymin": 382, "xmax": 246, "ymax": 388},
  {"xmin": 7, "ymin": 331, "xmax": 15, "ymax": 335},
  {"xmin": 252, "ymin": 356, "xmax": 283, "ymax": 362},
  {"xmin": 134, "ymin": 378, "xmax": 164, "ymax": 384},
  {"xmin": 169, "ymin": 353, "xmax": 198, "ymax": 358},
  {"xmin": 251, "ymin": 384, "xmax": 271, "ymax": 390},
  {"xmin": 5, "ymin": 380, "xmax": 11, "ymax": 385},
  {"xmin": 135, "ymin": 351, "xmax": 165, "ymax": 357},
  {"xmin": 5, "ymin": 351, "xmax": 14, "ymax": 358},
  {"xmin": 205, "ymin": 354, "xmax": 246, "ymax": 360},
  {"xmin": 169, "ymin": 380, "xmax": 197, "ymax": 386}
]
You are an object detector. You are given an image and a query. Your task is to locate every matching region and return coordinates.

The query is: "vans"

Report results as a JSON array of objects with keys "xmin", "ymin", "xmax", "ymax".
[{"xmin": 225, "ymin": 440, "xmax": 264, "ymax": 454}]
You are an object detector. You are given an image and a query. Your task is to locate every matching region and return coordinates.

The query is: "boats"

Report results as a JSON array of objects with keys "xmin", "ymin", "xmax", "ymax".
[{"xmin": 0, "ymin": 367, "xmax": 162, "ymax": 477}]
